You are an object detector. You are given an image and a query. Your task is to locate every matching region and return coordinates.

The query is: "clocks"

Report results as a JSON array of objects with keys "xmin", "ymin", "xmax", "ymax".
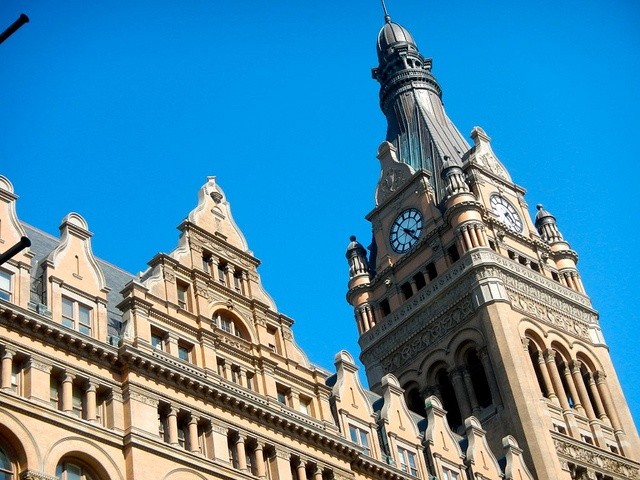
[
  {"xmin": 489, "ymin": 191, "xmax": 523, "ymax": 234},
  {"xmin": 389, "ymin": 208, "xmax": 424, "ymax": 254}
]
